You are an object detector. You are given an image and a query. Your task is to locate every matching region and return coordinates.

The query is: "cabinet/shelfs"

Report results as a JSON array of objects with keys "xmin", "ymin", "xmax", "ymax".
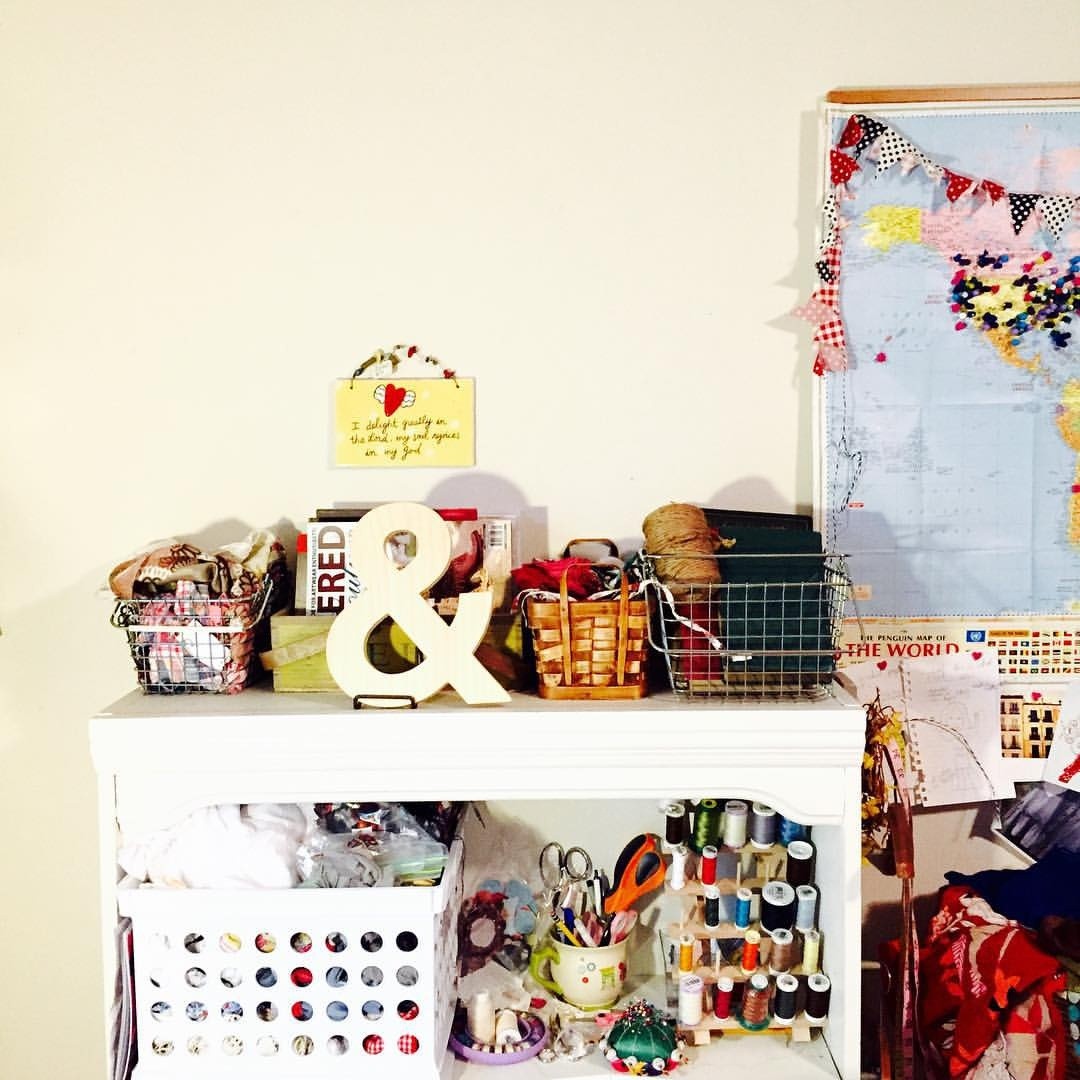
[{"xmin": 87, "ymin": 682, "xmax": 866, "ymax": 1080}]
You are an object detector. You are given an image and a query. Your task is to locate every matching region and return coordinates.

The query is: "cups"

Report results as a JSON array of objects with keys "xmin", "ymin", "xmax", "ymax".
[{"xmin": 530, "ymin": 932, "xmax": 629, "ymax": 1012}]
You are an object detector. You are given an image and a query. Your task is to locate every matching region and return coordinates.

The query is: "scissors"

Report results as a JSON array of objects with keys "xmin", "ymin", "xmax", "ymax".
[
  {"xmin": 607, "ymin": 910, "xmax": 638, "ymax": 945},
  {"xmin": 539, "ymin": 840, "xmax": 588, "ymax": 889}
]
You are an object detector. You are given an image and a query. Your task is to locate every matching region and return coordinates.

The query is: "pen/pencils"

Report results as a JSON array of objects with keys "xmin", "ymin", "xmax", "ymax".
[{"xmin": 550, "ymin": 869, "xmax": 604, "ymax": 947}]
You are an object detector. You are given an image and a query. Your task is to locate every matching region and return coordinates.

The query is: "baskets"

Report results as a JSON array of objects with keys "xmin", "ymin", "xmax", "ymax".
[
  {"xmin": 111, "ymin": 566, "xmax": 274, "ymax": 692},
  {"xmin": 636, "ymin": 543, "xmax": 850, "ymax": 704},
  {"xmin": 111, "ymin": 802, "xmax": 477, "ymax": 1078},
  {"xmin": 520, "ymin": 532, "xmax": 654, "ymax": 698}
]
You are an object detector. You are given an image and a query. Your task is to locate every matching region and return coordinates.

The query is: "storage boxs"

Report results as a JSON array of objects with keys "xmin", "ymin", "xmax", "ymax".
[
  {"xmin": 268, "ymin": 607, "xmax": 525, "ymax": 694},
  {"xmin": 115, "ymin": 825, "xmax": 466, "ymax": 1077}
]
